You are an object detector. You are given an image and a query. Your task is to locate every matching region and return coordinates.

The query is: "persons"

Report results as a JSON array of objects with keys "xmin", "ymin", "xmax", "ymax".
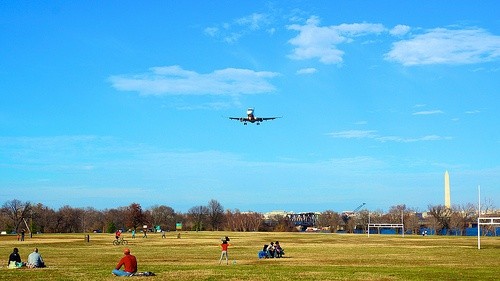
[
  {"xmin": 132, "ymin": 229, "xmax": 135, "ymax": 238},
  {"xmin": 422, "ymin": 229, "xmax": 428, "ymax": 236},
  {"xmin": 162, "ymin": 230, "xmax": 166, "ymax": 238},
  {"xmin": 28, "ymin": 247, "xmax": 46, "ymax": 268},
  {"xmin": 116, "ymin": 229, "xmax": 122, "ymax": 244},
  {"xmin": 7, "ymin": 248, "xmax": 22, "ymax": 268},
  {"xmin": 275, "ymin": 241, "xmax": 285, "ymax": 257},
  {"xmin": 21, "ymin": 230, "xmax": 25, "ymax": 241},
  {"xmin": 17, "ymin": 234, "xmax": 20, "ymax": 241},
  {"xmin": 219, "ymin": 240, "xmax": 231, "ymax": 265},
  {"xmin": 143, "ymin": 229, "xmax": 147, "ymax": 238},
  {"xmin": 266, "ymin": 242, "xmax": 275, "ymax": 258},
  {"xmin": 111, "ymin": 248, "xmax": 138, "ymax": 277}
]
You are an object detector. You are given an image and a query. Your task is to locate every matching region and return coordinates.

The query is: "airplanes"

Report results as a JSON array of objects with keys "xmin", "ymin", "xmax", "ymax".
[{"xmin": 229, "ymin": 108, "xmax": 276, "ymax": 125}]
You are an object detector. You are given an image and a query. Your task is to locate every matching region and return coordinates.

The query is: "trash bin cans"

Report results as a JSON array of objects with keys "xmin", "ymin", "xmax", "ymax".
[
  {"xmin": 85, "ymin": 234, "xmax": 89, "ymax": 242},
  {"xmin": 177, "ymin": 233, "xmax": 180, "ymax": 238}
]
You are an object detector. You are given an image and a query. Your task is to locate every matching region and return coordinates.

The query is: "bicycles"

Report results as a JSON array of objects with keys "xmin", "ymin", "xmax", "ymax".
[{"xmin": 113, "ymin": 236, "xmax": 128, "ymax": 246}]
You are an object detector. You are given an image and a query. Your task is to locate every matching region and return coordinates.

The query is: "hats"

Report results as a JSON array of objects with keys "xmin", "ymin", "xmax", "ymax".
[
  {"xmin": 13, "ymin": 248, "xmax": 19, "ymax": 252},
  {"xmin": 124, "ymin": 248, "xmax": 130, "ymax": 254},
  {"xmin": 270, "ymin": 242, "xmax": 274, "ymax": 244},
  {"xmin": 275, "ymin": 241, "xmax": 279, "ymax": 244}
]
